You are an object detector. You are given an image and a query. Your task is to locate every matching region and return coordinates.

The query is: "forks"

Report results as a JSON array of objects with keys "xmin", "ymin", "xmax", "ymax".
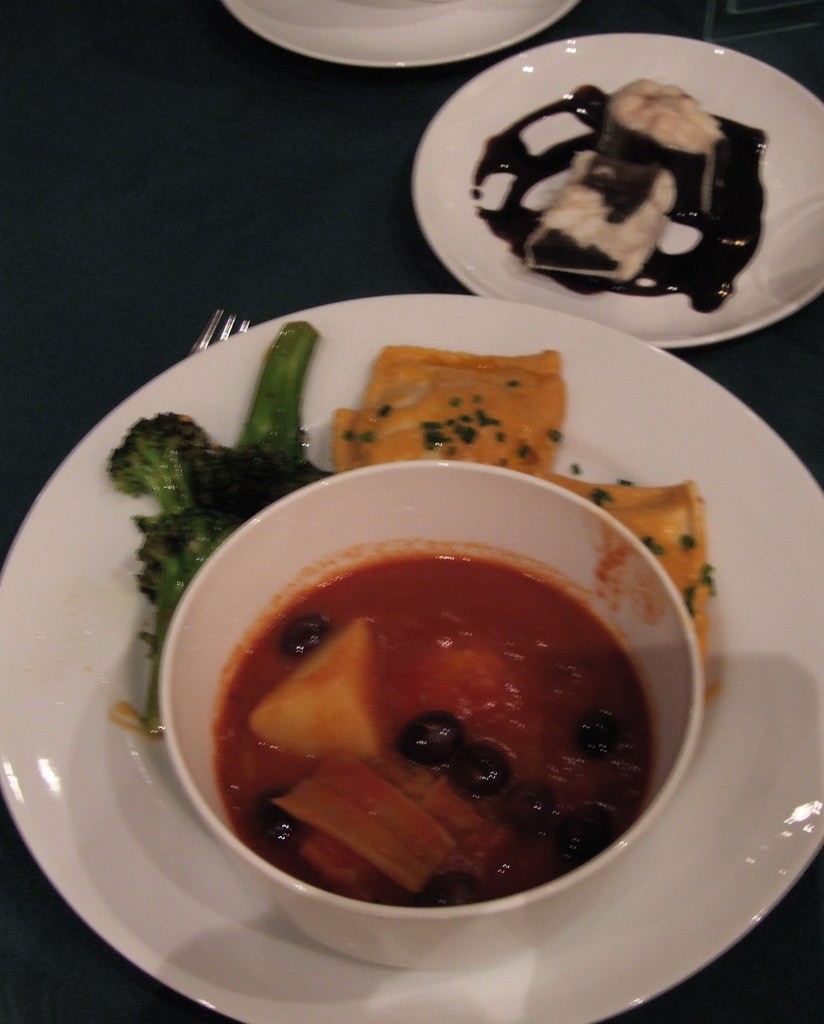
[{"xmin": 185, "ymin": 309, "xmax": 250, "ymax": 357}]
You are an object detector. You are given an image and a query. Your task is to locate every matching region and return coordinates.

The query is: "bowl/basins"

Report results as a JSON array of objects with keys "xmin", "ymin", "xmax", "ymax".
[{"xmin": 158, "ymin": 459, "xmax": 705, "ymax": 969}]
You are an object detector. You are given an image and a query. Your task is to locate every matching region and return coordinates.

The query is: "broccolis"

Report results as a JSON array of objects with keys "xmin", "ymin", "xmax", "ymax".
[{"xmin": 106, "ymin": 321, "xmax": 342, "ymax": 735}]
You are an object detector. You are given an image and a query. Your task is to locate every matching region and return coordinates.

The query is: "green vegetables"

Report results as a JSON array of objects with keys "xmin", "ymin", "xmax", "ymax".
[{"xmin": 341, "ymin": 393, "xmax": 716, "ymax": 620}]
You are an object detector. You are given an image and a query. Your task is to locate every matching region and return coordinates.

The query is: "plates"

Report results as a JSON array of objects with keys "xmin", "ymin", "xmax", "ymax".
[
  {"xmin": 411, "ymin": 33, "xmax": 824, "ymax": 347},
  {"xmin": 221, "ymin": 0, "xmax": 580, "ymax": 68},
  {"xmin": 0, "ymin": 289, "xmax": 824, "ymax": 1024}
]
